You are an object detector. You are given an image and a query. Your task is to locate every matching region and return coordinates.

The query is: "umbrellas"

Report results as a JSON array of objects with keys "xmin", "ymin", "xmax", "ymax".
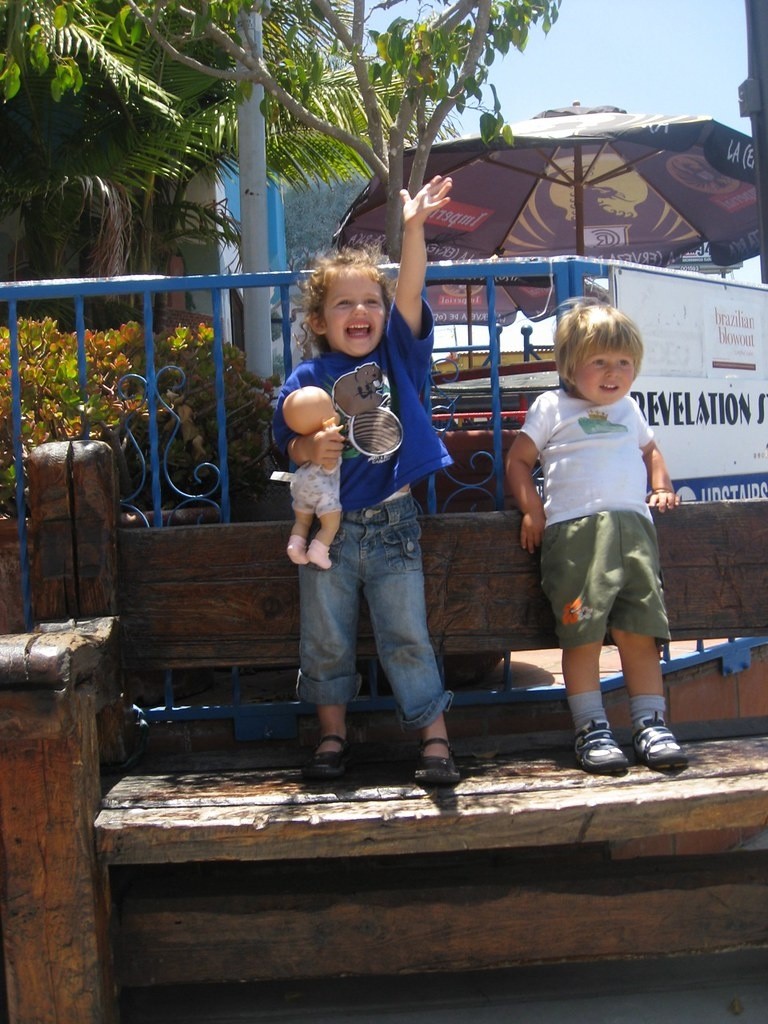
[{"xmin": 330, "ymin": 98, "xmax": 761, "ymax": 267}]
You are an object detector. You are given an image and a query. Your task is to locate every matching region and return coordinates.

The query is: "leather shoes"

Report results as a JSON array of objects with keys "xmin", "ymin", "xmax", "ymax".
[
  {"xmin": 414, "ymin": 737, "xmax": 461, "ymax": 782},
  {"xmin": 302, "ymin": 734, "xmax": 358, "ymax": 778}
]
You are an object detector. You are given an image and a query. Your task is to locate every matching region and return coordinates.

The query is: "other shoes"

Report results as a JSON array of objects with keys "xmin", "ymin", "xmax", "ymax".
[
  {"xmin": 573, "ymin": 719, "xmax": 629, "ymax": 774},
  {"xmin": 631, "ymin": 711, "xmax": 689, "ymax": 769}
]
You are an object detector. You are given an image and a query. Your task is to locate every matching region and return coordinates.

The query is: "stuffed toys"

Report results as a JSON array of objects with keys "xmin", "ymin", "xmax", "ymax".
[{"xmin": 270, "ymin": 385, "xmax": 344, "ymax": 570}]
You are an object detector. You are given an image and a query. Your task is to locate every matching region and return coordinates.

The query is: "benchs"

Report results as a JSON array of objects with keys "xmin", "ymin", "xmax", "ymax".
[{"xmin": 0, "ymin": 439, "xmax": 768, "ymax": 1024}]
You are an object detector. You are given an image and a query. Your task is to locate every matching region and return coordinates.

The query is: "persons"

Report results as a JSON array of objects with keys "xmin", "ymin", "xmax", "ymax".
[
  {"xmin": 271, "ymin": 174, "xmax": 460, "ymax": 786},
  {"xmin": 504, "ymin": 295, "xmax": 689, "ymax": 775}
]
